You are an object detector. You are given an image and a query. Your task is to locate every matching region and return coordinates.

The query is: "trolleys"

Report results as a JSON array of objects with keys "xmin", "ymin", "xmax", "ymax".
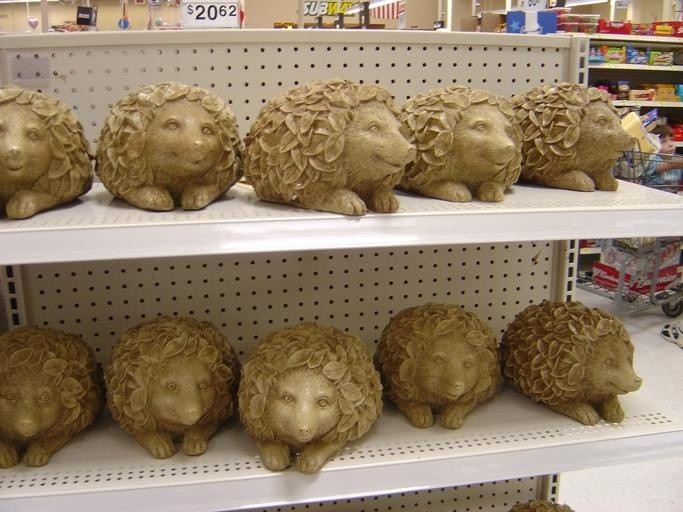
[{"xmin": 576, "ymin": 150, "xmax": 683, "ymax": 319}]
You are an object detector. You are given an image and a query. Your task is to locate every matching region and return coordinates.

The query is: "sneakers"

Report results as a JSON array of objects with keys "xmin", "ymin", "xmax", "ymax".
[{"xmin": 660, "ymin": 321, "xmax": 681, "ymax": 347}]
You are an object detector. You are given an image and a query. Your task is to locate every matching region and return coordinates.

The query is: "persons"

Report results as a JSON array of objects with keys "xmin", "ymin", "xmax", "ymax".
[{"xmin": 639, "ymin": 125, "xmax": 683, "ymax": 188}]
